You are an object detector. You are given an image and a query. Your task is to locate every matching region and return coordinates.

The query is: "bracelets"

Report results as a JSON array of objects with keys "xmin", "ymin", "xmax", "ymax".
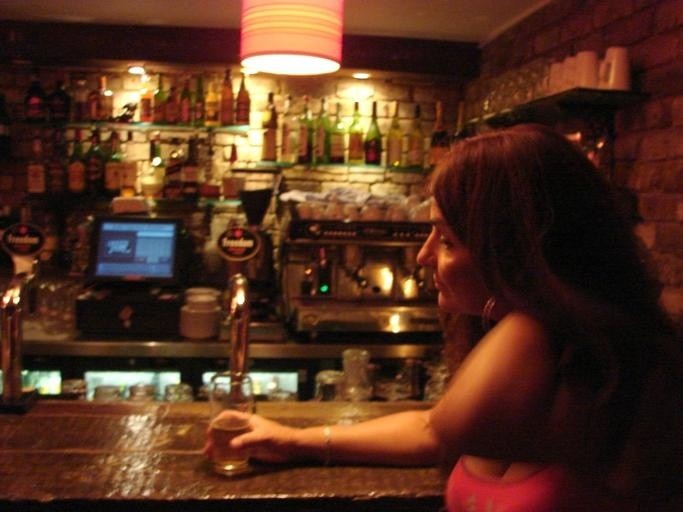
[{"xmin": 321, "ymin": 425, "xmax": 331, "ymax": 467}]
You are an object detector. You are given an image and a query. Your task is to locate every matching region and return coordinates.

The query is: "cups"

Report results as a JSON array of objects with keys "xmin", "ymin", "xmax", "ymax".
[
  {"xmin": 30, "ymin": 271, "xmax": 83, "ymax": 334},
  {"xmin": 538, "ymin": 45, "xmax": 630, "ymax": 94},
  {"xmin": 207, "ymin": 373, "xmax": 252, "ymax": 477}
]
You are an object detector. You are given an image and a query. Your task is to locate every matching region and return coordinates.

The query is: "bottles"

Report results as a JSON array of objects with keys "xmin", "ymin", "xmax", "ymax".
[
  {"xmin": 27, "ymin": 69, "xmax": 253, "ymax": 128},
  {"xmin": 58, "ymin": 346, "xmax": 451, "ymax": 403},
  {"xmin": 28, "ymin": 129, "xmax": 247, "ymax": 201},
  {"xmin": 178, "ymin": 286, "xmax": 223, "ymax": 341},
  {"xmin": 259, "ymin": 92, "xmax": 467, "ymax": 163},
  {"xmin": 459, "ymin": 68, "xmax": 538, "ymax": 115}
]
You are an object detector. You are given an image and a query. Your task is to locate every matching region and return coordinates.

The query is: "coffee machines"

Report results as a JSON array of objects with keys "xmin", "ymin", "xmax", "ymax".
[{"xmin": 280, "ymin": 219, "xmax": 448, "ymax": 346}]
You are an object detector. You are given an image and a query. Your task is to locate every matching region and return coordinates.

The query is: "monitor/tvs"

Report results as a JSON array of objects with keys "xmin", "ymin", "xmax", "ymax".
[{"xmin": 89, "ymin": 217, "xmax": 179, "ymax": 282}]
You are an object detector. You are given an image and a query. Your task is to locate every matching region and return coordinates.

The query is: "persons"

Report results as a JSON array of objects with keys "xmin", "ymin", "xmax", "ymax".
[{"xmin": 206, "ymin": 125, "xmax": 682, "ymax": 508}]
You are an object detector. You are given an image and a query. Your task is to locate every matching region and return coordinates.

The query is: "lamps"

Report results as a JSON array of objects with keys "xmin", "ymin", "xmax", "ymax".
[{"xmin": 239, "ymin": 0, "xmax": 344, "ymax": 76}]
